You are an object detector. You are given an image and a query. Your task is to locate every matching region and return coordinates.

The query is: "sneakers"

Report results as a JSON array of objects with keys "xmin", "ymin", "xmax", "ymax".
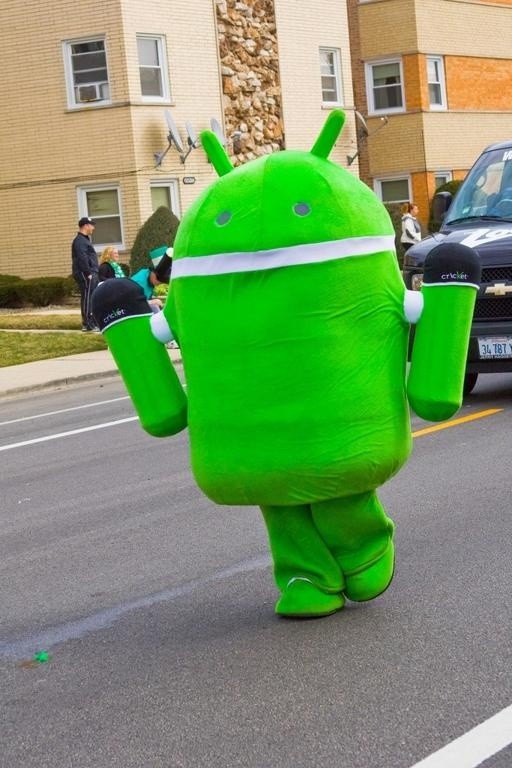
[{"xmin": 82, "ymin": 325, "xmax": 101, "ymax": 334}]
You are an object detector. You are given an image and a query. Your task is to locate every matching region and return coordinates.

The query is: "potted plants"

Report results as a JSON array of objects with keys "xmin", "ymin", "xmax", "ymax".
[{"xmin": 151, "ymin": 283, "xmax": 170, "ymax": 303}]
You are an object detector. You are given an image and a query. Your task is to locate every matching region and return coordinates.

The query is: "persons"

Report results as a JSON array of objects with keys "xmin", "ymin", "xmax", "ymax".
[
  {"xmin": 72, "ymin": 216, "xmax": 102, "ymax": 331},
  {"xmin": 98, "ymin": 245, "xmax": 129, "ymax": 281},
  {"xmin": 131, "ymin": 267, "xmax": 160, "ymax": 302},
  {"xmin": 400, "ymin": 203, "xmax": 421, "ymax": 252}
]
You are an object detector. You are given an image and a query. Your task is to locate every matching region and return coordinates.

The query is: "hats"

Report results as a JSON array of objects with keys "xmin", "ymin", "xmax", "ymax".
[
  {"xmin": 149, "ymin": 266, "xmax": 162, "ymax": 280},
  {"xmin": 79, "ymin": 217, "xmax": 97, "ymax": 226}
]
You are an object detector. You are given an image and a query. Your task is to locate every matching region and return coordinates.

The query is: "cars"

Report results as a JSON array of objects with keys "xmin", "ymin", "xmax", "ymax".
[{"xmin": 403, "ymin": 140, "xmax": 512, "ymax": 398}]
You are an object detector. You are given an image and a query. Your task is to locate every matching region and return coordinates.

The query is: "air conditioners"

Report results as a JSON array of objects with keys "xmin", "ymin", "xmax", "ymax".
[{"xmin": 77, "ymin": 84, "xmax": 102, "ymax": 103}]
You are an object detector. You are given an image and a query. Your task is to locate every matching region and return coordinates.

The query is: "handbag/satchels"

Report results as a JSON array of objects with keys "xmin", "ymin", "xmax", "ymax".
[{"xmin": 405, "ymin": 223, "xmax": 419, "ymax": 239}]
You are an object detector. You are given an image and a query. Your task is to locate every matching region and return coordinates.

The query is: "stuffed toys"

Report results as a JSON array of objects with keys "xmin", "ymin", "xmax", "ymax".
[{"xmin": 88, "ymin": 107, "xmax": 480, "ymax": 617}]
